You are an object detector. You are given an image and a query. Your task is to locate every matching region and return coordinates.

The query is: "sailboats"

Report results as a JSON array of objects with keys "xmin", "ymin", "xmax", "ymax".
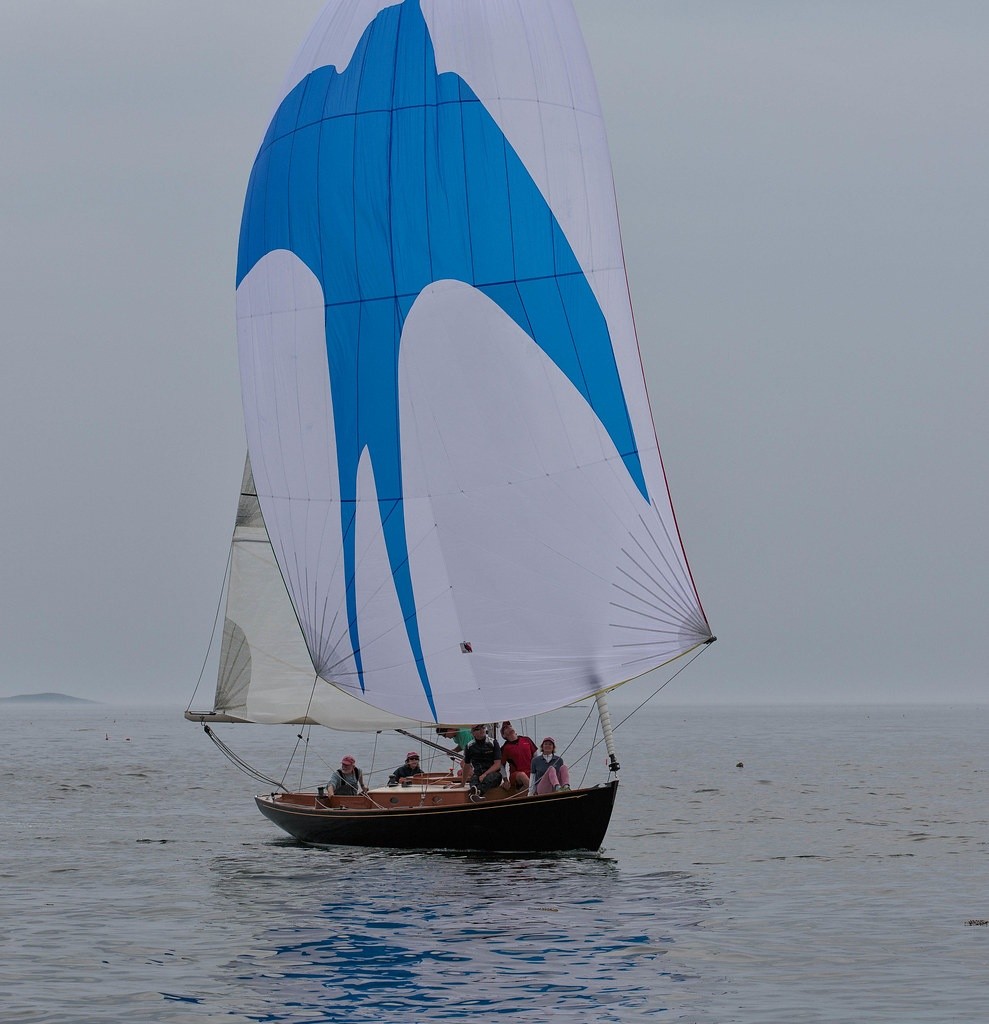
[{"xmin": 184, "ymin": 1, "xmax": 718, "ymax": 854}]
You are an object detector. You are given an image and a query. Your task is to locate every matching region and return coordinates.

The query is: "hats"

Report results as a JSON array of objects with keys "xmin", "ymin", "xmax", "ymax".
[
  {"xmin": 340, "ymin": 755, "xmax": 355, "ymax": 765},
  {"xmin": 471, "ymin": 724, "xmax": 484, "ymax": 729},
  {"xmin": 407, "ymin": 752, "xmax": 420, "ymax": 757},
  {"xmin": 543, "ymin": 736, "xmax": 555, "ymax": 744}
]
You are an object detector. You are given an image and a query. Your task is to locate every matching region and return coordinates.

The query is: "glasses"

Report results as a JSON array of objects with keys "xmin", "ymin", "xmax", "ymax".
[
  {"xmin": 409, "ymin": 757, "xmax": 419, "ymax": 760},
  {"xmin": 475, "ymin": 727, "xmax": 486, "ymax": 730},
  {"xmin": 503, "ymin": 725, "xmax": 512, "ymax": 729}
]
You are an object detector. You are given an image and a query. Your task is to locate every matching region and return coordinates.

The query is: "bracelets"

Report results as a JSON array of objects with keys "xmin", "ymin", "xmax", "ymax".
[{"xmin": 361, "ymin": 785, "xmax": 364, "ymax": 787}]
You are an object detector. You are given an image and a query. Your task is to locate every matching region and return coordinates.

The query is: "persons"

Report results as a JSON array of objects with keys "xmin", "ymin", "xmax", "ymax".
[
  {"xmin": 327, "ymin": 754, "xmax": 369, "ymax": 799},
  {"xmin": 437, "ymin": 728, "xmax": 475, "ymax": 777},
  {"xmin": 452, "ymin": 725, "xmax": 502, "ymax": 802},
  {"xmin": 387, "ymin": 752, "xmax": 424, "ymax": 787},
  {"xmin": 500, "ymin": 721, "xmax": 538, "ymax": 790},
  {"xmin": 527, "ymin": 737, "xmax": 571, "ymax": 796}
]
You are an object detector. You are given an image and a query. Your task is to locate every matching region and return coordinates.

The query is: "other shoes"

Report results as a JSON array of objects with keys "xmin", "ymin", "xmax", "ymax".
[
  {"xmin": 555, "ymin": 784, "xmax": 572, "ymax": 792},
  {"xmin": 470, "ymin": 795, "xmax": 486, "ymax": 803},
  {"xmin": 469, "ymin": 785, "xmax": 477, "ymax": 796}
]
